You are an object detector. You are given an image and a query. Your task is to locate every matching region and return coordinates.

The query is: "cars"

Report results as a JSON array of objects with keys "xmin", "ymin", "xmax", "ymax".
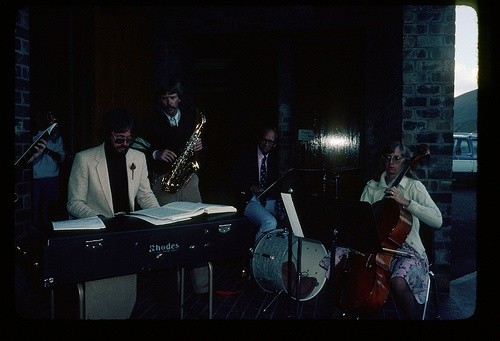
[{"xmin": 452, "ymin": 131, "xmax": 478, "ymax": 177}]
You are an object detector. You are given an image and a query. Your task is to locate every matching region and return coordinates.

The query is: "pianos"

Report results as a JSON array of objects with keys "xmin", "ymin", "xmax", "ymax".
[{"xmin": 24, "ymin": 210, "xmax": 259, "ymax": 290}]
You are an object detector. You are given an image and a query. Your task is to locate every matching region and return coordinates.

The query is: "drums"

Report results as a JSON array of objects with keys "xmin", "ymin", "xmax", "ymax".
[{"xmin": 251, "ymin": 228, "xmax": 329, "ymax": 302}]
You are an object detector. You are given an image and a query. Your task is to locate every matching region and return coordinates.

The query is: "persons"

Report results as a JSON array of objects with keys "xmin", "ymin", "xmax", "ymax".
[
  {"xmin": 131, "ymin": 84, "xmax": 221, "ymax": 317},
  {"xmin": 31, "ymin": 112, "xmax": 66, "ymax": 246},
  {"xmin": 359, "ymin": 141, "xmax": 443, "ymax": 321},
  {"xmin": 67, "ymin": 107, "xmax": 161, "ymax": 221},
  {"xmin": 232, "ymin": 129, "xmax": 287, "ymax": 246},
  {"xmin": 14, "ymin": 138, "xmax": 47, "ymax": 168}
]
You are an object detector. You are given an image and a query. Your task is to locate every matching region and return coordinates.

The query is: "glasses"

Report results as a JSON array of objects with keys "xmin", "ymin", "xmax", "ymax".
[
  {"xmin": 382, "ymin": 154, "xmax": 402, "ymax": 163},
  {"xmin": 112, "ymin": 133, "xmax": 133, "ymax": 143},
  {"xmin": 259, "ymin": 137, "xmax": 277, "ymax": 147}
]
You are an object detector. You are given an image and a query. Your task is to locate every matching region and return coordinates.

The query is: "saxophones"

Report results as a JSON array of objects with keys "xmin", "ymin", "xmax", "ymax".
[{"xmin": 158, "ymin": 101, "xmax": 212, "ymax": 194}]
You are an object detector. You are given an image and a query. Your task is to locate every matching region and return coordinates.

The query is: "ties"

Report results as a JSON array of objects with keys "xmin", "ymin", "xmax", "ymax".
[
  {"xmin": 261, "ymin": 155, "xmax": 266, "ymax": 182},
  {"xmin": 170, "ymin": 117, "xmax": 176, "ymax": 126}
]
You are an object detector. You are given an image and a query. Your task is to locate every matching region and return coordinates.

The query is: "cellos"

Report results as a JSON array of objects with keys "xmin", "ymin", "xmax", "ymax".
[{"xmin": 324, "ymin": 142, "xmax": 428, "ymax": 319}]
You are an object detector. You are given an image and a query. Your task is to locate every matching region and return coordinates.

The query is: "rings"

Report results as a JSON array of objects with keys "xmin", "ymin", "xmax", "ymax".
[{"xmin": 392, "ymin": 192, "xmax": 394, "ymax": 194}]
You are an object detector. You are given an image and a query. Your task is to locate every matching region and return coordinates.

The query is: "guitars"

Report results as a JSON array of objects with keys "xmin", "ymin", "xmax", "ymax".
[{"xmin": 13, "ymin": 119, "xmax": 62, "ymax": 166}]
